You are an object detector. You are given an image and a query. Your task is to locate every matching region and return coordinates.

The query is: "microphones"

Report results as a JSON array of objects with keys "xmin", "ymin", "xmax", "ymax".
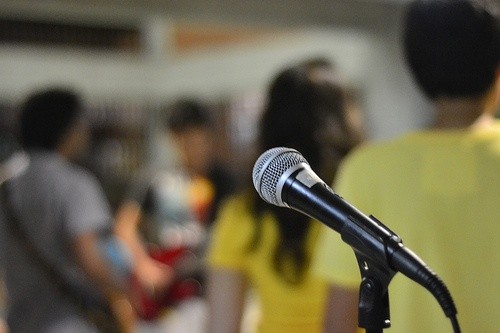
[{"xmin": 252, "ymin": 147, "xmax": 436, "ymax": 288}]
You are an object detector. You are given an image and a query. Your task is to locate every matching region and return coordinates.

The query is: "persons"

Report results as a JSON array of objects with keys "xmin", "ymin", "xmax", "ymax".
[
  {"xmin": 0, "ymin": 84, "xmax": 147, "ymax": 333},
  {"xmin": 305, "ymin": 1, "xmax": 500, "ymax": 332},
  {"xmin": 200, "ymin": 55, "xmax": 374, "ymax": 332},
  {"xmin": 111, "ymin": 94, "xmax": 244, "ymax": 333}
]
